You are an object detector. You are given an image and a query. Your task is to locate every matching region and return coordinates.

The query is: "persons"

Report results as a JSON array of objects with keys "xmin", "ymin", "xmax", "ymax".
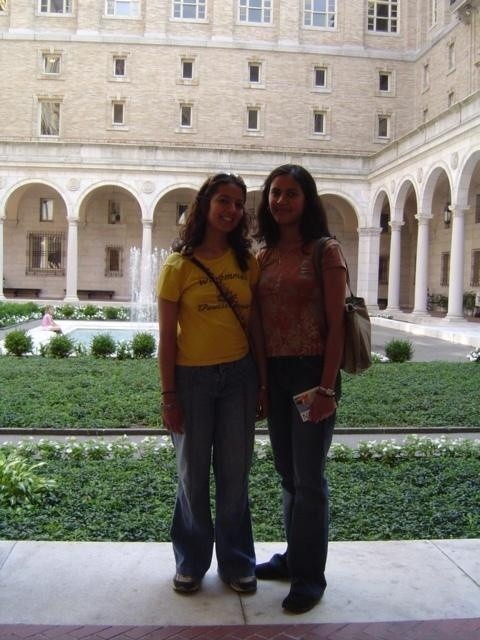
[
  {"xmin": 152, "ymin": 172, "xmax": 269, "ymax": 595},
  {"xmin": 250, "ymin": 165, "xmax": 349, "ymax": 614}
]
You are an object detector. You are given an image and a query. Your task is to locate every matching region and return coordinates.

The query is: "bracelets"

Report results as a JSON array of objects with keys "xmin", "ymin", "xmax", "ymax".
[
  {"xmin": 258, "ymin": 384, "xmax": 268, "ymax": 389},
  {"xmin": 161, "ymin": 391, "xmax": 177, "ymax": 395},
  {"xmin": 318, "ymin": 386, "xmax": 336, "ymax": 397}
]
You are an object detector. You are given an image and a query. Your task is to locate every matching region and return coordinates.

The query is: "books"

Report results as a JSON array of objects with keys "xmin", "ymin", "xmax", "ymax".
[{"xmin": 293, "ymin": 385, "xmax": 323, "ymax": 424}]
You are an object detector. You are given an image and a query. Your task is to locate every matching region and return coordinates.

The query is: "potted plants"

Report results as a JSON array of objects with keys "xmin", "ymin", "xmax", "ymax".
[
  {"xmin": 435, "ymin": 296, "xmax": 448, "ymax": 312},
  {"xmin": 464, "ymin": 291, "xmax": 476, "ymax": 317}
]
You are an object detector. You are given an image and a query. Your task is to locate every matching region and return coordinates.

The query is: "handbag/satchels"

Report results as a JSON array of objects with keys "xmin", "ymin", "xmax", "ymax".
[{"xmin": 315, "ymin": 237, "xmax": 372, "ymax": 374}]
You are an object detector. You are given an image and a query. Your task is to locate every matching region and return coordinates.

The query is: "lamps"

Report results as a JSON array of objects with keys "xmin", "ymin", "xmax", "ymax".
[{"xmin": 443, "ymin": 201, "xmax": 452, "ymax": 224}]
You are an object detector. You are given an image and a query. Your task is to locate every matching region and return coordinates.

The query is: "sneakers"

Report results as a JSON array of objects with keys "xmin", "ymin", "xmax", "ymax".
[
  {"xmin": 218, "ymin": 569, "xmax": 257, "ymax": 592},
  {"xmin": 255, "ymin": 554, "xmax": 288, "ymax": 579},
  {"xmin": 282, "ymin": 580, "xmax": 327, "ymax": 613},
  {"xmin": 171, "ymin": 572, "xmax": 201, "ymax": 592}
]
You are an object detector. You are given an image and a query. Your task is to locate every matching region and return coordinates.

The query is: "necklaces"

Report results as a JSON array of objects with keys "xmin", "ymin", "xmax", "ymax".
[{"xmin": 274, "ymin": 238, "xmax": 301, "ymax": 265}]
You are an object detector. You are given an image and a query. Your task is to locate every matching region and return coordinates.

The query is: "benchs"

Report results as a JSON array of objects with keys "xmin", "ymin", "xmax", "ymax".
[
  {"xmin": 77, "ymin": 290, "xmax": 116, "ymax": 300},
  {"xmin": 4, "ymin": 288, "xmax": 42, "ymax": 298}
]
